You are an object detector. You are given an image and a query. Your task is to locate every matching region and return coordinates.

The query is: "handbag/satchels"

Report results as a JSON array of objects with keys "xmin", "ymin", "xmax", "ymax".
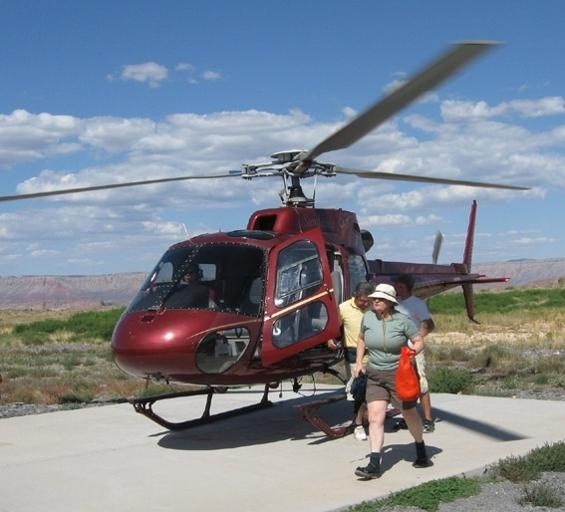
[{"xmin": 395, "ymin": 346, "xmax": 420, "ymax": 401}]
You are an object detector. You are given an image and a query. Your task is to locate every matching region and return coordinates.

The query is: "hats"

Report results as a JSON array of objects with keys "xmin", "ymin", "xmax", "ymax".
[{"xmin": 367, "ymin": 283, "xmax": 399, "ymax": 306}]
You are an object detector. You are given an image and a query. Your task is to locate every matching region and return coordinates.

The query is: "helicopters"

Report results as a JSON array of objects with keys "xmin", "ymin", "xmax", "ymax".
[{"xmin": 0, "ymin": 40, "xmax": 530, "ymax": 438}]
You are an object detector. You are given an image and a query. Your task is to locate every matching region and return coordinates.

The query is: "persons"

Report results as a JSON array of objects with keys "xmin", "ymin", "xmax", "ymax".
[
  {"xmin": 326, "ymin": 281, "xmax": 376, "ymax": 442},
  {"xmin": 394, "ymin": 273, "xmax": 436, "ymax": 434},
  {"xmin": 355, "ymin": 284, "xmax": 428, "ymax": 479}
]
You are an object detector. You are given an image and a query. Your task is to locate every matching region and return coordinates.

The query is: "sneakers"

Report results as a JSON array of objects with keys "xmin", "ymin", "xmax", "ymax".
[
  {"xmin": 353, "ymin": 425, "xmax": 368, "ymax": 441},
  {"xmin": 354, "ymin": 464, "xmax": 381, "ymax": 479},
  {"xmin": 413, "ymin": 455, "xmax": 428, "ymax": 469},
  {"xmin": 423, "ymin": 420, "xmax": 435, "ymax": 434}
]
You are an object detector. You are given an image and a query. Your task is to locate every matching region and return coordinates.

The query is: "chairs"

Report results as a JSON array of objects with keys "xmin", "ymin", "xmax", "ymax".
[{"xmin": 237, "ymin": 275, "xmax": 265, "ymax": 317}]
